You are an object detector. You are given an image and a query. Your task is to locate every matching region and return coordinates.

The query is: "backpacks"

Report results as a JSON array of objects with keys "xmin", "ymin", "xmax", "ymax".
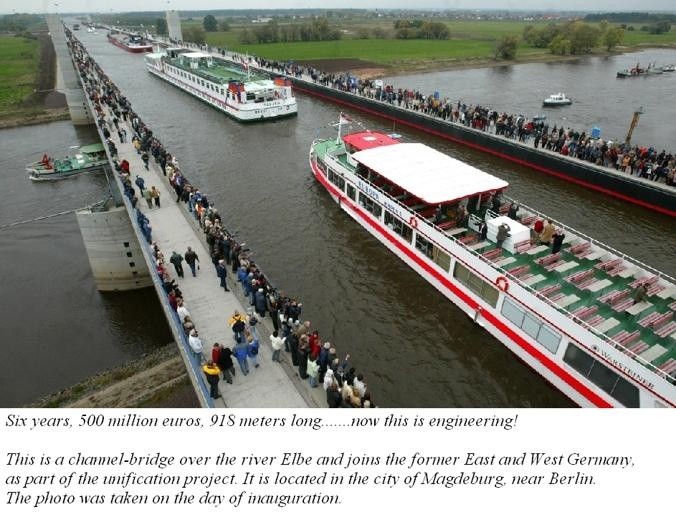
[{"xmin": 232, "ymin": 317, "xmax": 246, "ymax": 333}]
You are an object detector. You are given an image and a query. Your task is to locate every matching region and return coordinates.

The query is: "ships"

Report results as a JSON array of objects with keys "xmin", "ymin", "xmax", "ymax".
[
  {"xmin": 308, "ymin": 110, "xmax": 676, "ymax": 409},
  {"xmin": 143, "ymin": 46, "xmax": 298, "ymax": 124}
]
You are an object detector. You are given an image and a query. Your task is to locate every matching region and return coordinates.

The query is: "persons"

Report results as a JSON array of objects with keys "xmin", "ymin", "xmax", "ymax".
[{"xmin": 62, "ymin": 26, "xmax": 676, "ymax": 407}]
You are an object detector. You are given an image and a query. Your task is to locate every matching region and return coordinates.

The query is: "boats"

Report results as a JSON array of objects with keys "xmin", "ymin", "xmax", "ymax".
[
  {"xmin": 542, "ymin": 91, "xmax": 572, "ymax": 106},
  {"xmin": 616, "ymin": 61, "xmax": 676, "ymax": 78},
  {"xmin": 73, "ymin": 16, "xmax": 106, "ymax": 33},
  {"xmin": 24, "ymin": 142, "xmax": 112, "ymax": 184},
  {"xmin": 107, "ymin": 29, "xmax": 153, "ymax": 53}
]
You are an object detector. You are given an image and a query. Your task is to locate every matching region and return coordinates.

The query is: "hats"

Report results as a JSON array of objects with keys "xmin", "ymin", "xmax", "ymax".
[
  {"xmin": 258, "ymin": 288, "xmax": 264, "ymax": 293},
  {"xmin": 329, "ymin": 348, "xmax": 337, "ymax": 354},
  {"xmin": 252, "ymin": 279, "xmax": 256, "ymax": 286},
  {"xmin": 323, "ymin": 342, "xmax": 331, "ymax": 350},
  {"xmin": 175, "ymin": 297, "xmax": 183, "ymax": 306}
]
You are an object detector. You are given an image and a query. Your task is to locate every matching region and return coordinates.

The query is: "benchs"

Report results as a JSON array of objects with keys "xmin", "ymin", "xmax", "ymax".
[{"xmin": 355, "ymin": 166, "xmax": 676, "ymax": 384}]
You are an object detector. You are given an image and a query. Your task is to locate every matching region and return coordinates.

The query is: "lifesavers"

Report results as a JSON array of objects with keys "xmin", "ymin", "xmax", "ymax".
[
  {"xmin": 496, "ymin": 276, "xmax": 508, "ymax": 291},
  {"xmin": 408, "ymin": 216, "xmax": 417, "ymax": 228}
]
